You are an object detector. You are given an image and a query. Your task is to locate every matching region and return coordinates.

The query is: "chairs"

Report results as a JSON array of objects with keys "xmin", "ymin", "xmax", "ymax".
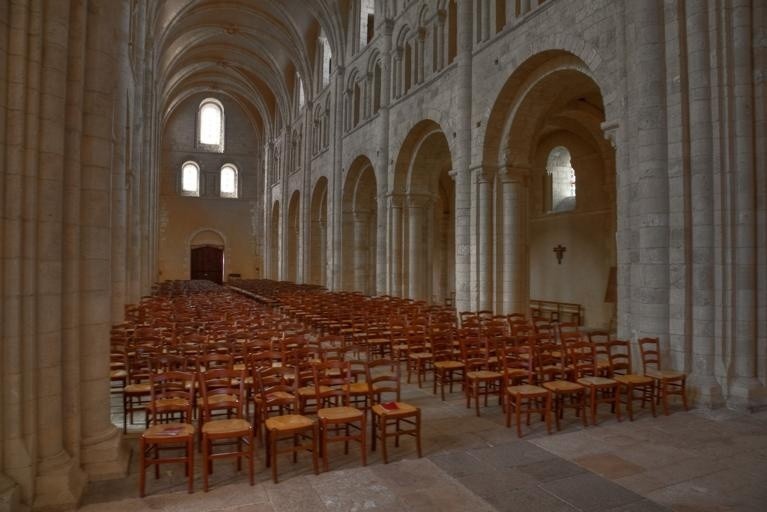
[
  {"xmin": 139, "ymin": 373, "xmax": 198, "ymax": 498},
  {"xmin": 317, "ymin": 363, "xmax": 367, "ymax": 472},
  {"xmin": 260, "ymin": 367, "xmax": 320, "ymax": 483},
  {"xmin": 197, "ymin": 368, "xmax": 255, "ymax": 490},
  {"xmin": 366, "ymin": 358, "xmax": 423, "ymax": 464}
]
